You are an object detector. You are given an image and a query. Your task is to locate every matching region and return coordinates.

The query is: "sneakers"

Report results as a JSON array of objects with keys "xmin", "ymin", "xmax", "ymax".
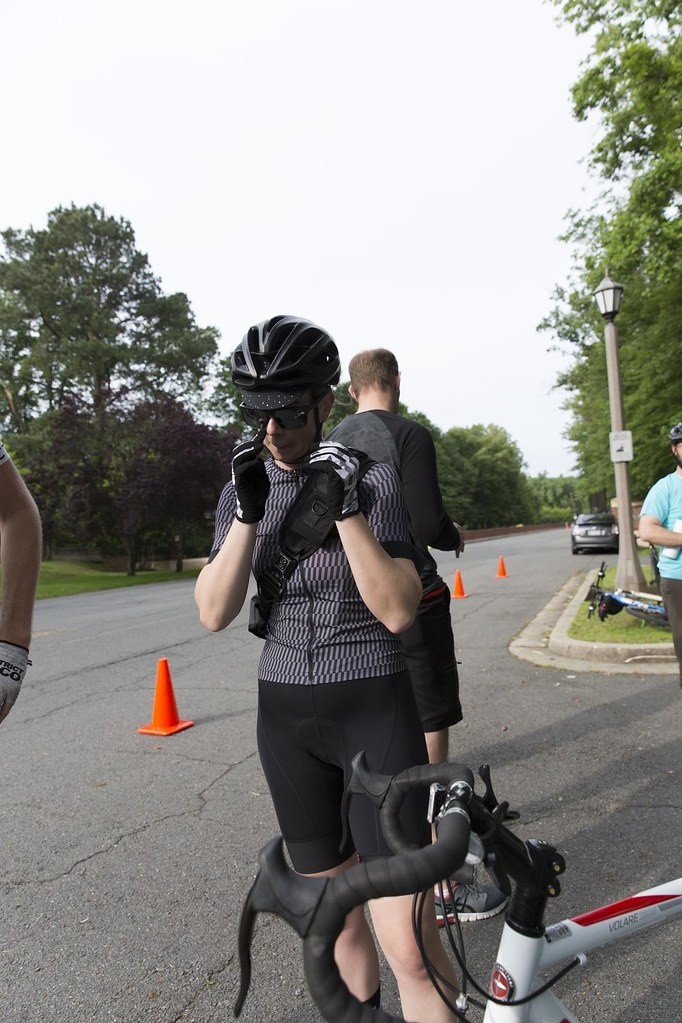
[{"xmin": 433, "ymin": 881, "xmax": 509, "ymax": 928}]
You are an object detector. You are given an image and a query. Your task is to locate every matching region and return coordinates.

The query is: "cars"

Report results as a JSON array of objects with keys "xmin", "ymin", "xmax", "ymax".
[{"xmin": 570, "ymin": 513, "xmax": 619, "ymax": 555}]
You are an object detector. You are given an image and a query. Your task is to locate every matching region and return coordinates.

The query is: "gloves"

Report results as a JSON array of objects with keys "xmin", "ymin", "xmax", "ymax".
[
  {"xmin": 0, "ymin": 640, "xmax": 33, "ymax": 725},
  {"xmin": 302, "ymin": 440, "xmax": 361, "ymax": 520},
  {"xmin": 231, "ymin": 427, "xmax": 271, "ymax": 524}
]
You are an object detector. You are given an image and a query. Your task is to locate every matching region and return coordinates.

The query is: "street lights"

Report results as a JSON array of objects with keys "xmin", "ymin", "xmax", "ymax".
[{"xmin": 590, "ymin": 267, "xmax": 652, "ymax": 601}]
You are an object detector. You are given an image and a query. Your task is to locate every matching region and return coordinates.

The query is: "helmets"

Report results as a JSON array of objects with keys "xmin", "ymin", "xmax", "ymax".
[
  {"xmin": 230, "ymin": 315, "xmax": 341, "ymax": 392},
  {"xmin": 668, "ymin": 423, "xmax": 682, "ymax": 440}
]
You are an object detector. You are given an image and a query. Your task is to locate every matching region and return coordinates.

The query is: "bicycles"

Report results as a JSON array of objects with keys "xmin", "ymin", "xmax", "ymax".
[
  {"xmin": 587, "ymin": 561, "xmax": 670, "ymax": 626},
  {"xmin": 232, "ymin": 750, "xmax": 682, "ymax": 1023}
]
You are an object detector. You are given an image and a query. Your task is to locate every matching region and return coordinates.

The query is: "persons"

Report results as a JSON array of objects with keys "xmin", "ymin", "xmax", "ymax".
[
  {"xmin": 639, "ymin": 422, "xmax": 682, "ymax": 685},
  {"xmin": 322, "ymin": 348, "xmax": 508, "ymax": 921},
  {"xmin": 193, "ymin": 315, "xmax": 461, "ymax": 1023},
  {"xmin": 0, "ymin": 440, "xmax": 42, "ymax": 722}
]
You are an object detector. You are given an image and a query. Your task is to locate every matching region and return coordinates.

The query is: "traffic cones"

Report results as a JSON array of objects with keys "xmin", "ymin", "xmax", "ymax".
[
  {"xmin": 138, "ymin": 658, "xmax": 196, "ymax": 737},
  {"xmin": 450, "ymin": 569, "xmax": 470, "ymax": 599},
  {"xmin": 495, "ymin": 555, "xmax": 510, "ymax": 579}
]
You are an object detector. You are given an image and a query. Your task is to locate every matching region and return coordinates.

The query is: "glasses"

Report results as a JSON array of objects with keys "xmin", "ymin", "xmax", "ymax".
[{"xmin": 239, "ymin": 386, "xmax": 325, "ymax": 430}]
